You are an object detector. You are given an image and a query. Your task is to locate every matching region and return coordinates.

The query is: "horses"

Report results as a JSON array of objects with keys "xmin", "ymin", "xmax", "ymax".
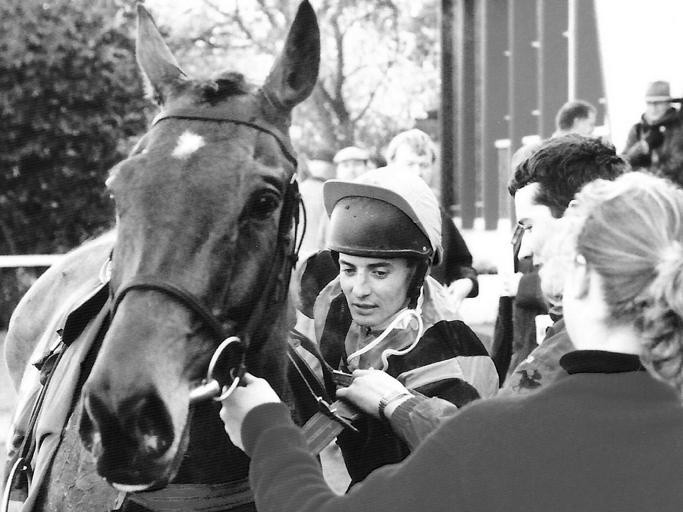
[{"xmin": 0, "ymin": 0, "xmax": 337, "ymax": 512}]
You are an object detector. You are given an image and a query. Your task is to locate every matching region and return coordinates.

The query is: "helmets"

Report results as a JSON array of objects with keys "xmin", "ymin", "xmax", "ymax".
[{"xmin": 323, "ymin": 164, "xmax": 443, "ymax": 268}]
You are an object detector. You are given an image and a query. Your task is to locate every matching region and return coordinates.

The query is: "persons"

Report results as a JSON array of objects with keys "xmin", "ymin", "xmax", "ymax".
[
  {"xmin": 552, "ymin": 101, "xmax": 598, "ymax": 136},
  {"xmin": 314, "ymin": 141, "xmax": 381, "ymax": 252},
  {"xmin": 310, "ymin": 158, "xmax": 500, "ymax": 495},
  {"xmin": 379, "ymin": 128, "xmax": 481, "ymax": 304},
  {"xmin": 619, "ymin": 81, "xmax": 683, "ymax": 192},
  {"xmin": 499, "ymin": 131, "xmax": 639, "ymax": 392},
  {"xmin": 213, "ymin": 169, "xmax": 683, "ymax": 512}
]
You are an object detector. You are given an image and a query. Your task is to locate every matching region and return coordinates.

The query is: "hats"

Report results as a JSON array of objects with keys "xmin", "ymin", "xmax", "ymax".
[{"xmin": 333, "ymin": 147, "xmax": 369, "ymax": 163}]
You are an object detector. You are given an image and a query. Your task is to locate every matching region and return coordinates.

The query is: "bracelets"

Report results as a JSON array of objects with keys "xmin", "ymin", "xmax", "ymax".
[{"xmin": 376, "ymin": 389, "xmax": 411, "ymax": 419}]
